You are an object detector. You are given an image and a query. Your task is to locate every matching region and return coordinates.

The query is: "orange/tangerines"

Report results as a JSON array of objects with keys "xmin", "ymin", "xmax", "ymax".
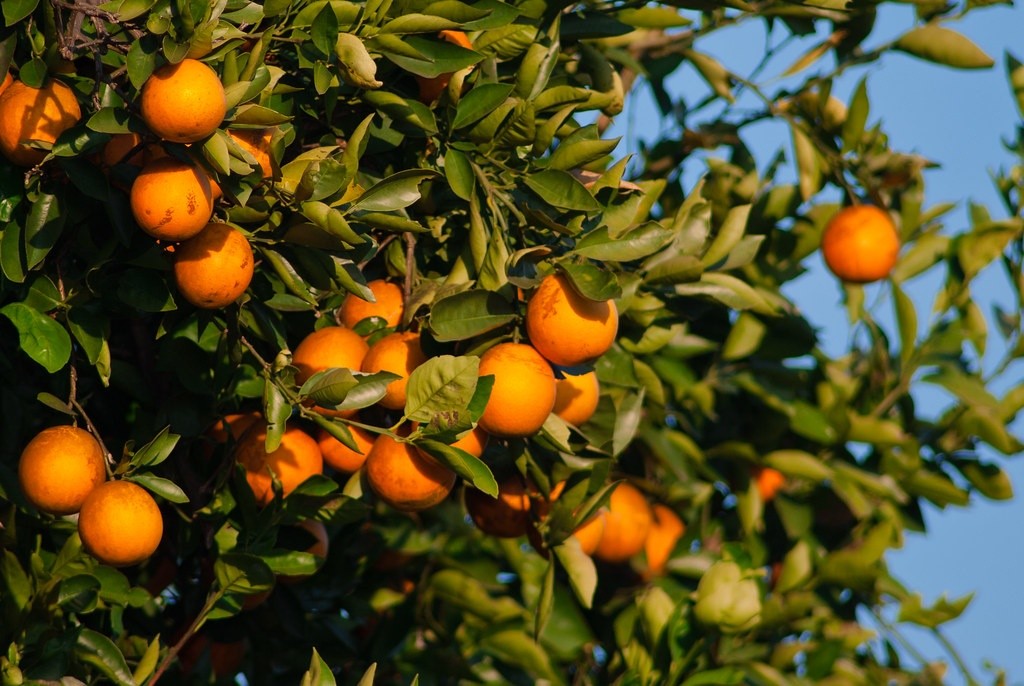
[{"xmin": 0, "ymin": 30, "xmax": 897, "ymax": 578}]
